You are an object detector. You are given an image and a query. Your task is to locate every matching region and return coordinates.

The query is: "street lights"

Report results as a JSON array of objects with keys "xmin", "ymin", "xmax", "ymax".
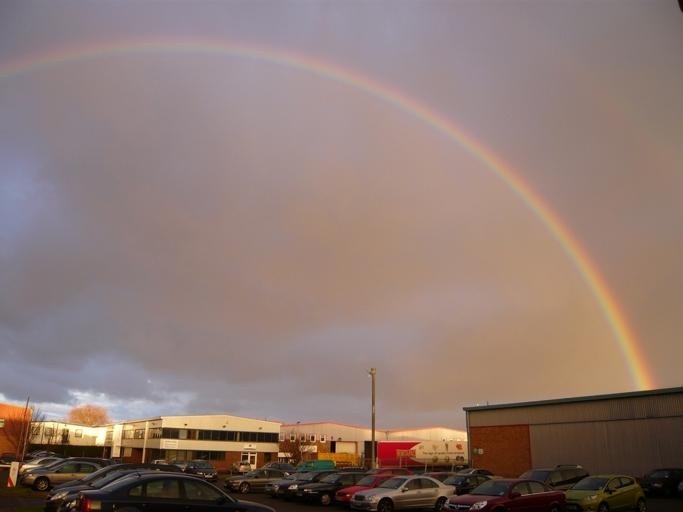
[{"xmin": 363, "ymin": 364, "xmax": 378, "ymax": 469}]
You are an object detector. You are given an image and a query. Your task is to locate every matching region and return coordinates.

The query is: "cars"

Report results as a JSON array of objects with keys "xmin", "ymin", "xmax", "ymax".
[
  {"xmin": 640, "ymin": 469, "xmax": 683, "ymax": 502},
  {"xmin": 76, "ymin": 473, "xmax": 277, "ymax": 512},
  {"xmin": 18, "ymin": 448, "xmax": 218, "ymax": 512},
  {"xmin": 564, "ymin": 474, "xmax": 647, "ymax": 512},
  {"xmin": 230, "ymin": 462, "xmax": 251, "ymax": 473},
  {"xmin": 349, "ymin": 475, "xmax": 457, "ymax": 512},
  {"xmin": 261, "ymin": 458, "xmax": 504, "ymax": 508},
  {"xmin": 443, "ymin": 478, "xmax": 567, "ymax": 512},
  {"xmin": 222, "ymin": 467, "xmax": 285, "ymax": 494}
]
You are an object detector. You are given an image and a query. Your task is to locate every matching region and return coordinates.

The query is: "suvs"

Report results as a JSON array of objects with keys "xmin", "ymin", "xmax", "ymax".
[{"xmin": 517, "ymin": 464, "xmax": 590, "ymax": 490}]
